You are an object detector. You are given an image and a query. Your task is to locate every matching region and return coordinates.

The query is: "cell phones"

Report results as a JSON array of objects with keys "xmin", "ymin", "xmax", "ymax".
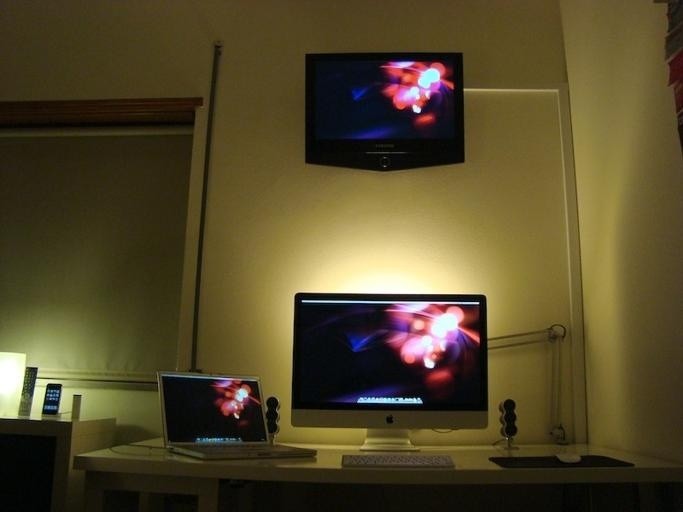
[{"xmin": 42, "ymin": 383, "xmax": 62, "ymax": 414}]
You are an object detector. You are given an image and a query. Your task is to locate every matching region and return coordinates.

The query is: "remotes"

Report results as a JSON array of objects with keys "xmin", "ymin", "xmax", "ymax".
[{"xmin": 17, "ymin": 367, "xmax": 37, "ymax": 416}]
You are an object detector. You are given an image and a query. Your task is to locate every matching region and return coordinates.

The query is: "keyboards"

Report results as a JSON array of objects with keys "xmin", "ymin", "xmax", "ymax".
[{"xmin": 343, "ymin": 453, "xmax": 455, "ymax": 468}]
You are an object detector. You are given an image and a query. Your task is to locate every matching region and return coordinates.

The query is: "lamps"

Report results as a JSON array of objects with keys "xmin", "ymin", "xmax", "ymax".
[{"xmin": 0, "ymin": 352, "xmax": 26, "ymax": 395}]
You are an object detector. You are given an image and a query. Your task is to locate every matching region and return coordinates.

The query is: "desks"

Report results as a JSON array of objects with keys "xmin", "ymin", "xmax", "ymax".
[{"xmin": 70, "ymin": 432, "xmax": 668, "ymax": 508}]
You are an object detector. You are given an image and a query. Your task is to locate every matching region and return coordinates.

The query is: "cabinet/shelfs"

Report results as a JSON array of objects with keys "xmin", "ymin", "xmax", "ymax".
[{"xmin": 0, "ymin": 414, "xmax": 118, "ymax": 511}]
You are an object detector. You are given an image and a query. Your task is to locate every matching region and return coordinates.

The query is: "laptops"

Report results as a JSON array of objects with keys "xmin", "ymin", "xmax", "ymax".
[{"xmin": 155, "ymin": 370, "xmax": 316, "ymax": 461}]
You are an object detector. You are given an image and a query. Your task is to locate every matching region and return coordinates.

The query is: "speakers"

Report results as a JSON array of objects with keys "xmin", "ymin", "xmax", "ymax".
[
  {"xmin": 500, "ymin": 399, "xmax": 518, "ymax": 450},
  {"xmin": 266, "ymin": 396, "xmax": 281, "ymax": 443}
]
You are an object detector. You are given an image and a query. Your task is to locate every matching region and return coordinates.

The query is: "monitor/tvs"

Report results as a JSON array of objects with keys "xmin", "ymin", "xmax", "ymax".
[
  {"xmin": 305, "ymin": 52, "xmax": 464, "ymax": 170},
  {"xmin": 291, "ymin": 293, "xmax": 487, "ymax": 451}
]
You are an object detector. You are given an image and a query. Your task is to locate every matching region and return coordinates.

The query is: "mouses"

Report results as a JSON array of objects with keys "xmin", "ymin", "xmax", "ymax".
[{"xmin": 557, "ymin": 452, "xmax": 581, "ymax": 463}]
